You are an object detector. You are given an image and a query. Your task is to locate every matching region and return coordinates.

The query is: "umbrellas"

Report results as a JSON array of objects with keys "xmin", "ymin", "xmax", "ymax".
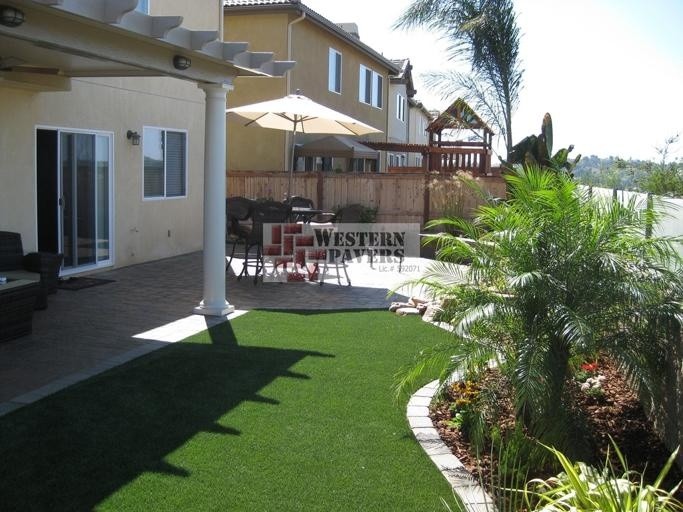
[{"xmin": 226, "ymin": 89, "xmax": 385, "ymax": 206}]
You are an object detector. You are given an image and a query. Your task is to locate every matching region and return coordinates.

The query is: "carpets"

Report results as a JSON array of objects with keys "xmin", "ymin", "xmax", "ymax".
[{"xmin": 55, "ymin": 275, "xmax": 116, "ymax": 291}]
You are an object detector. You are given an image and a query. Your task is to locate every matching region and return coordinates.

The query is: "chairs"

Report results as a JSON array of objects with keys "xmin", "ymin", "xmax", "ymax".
[
  {"xmin": 225, "ymin": 194, "xmax": 363, "ymax": 291},
  {"xmin": 0, "ymin": 230, "xmax": 51, "ymax": 311}
]
